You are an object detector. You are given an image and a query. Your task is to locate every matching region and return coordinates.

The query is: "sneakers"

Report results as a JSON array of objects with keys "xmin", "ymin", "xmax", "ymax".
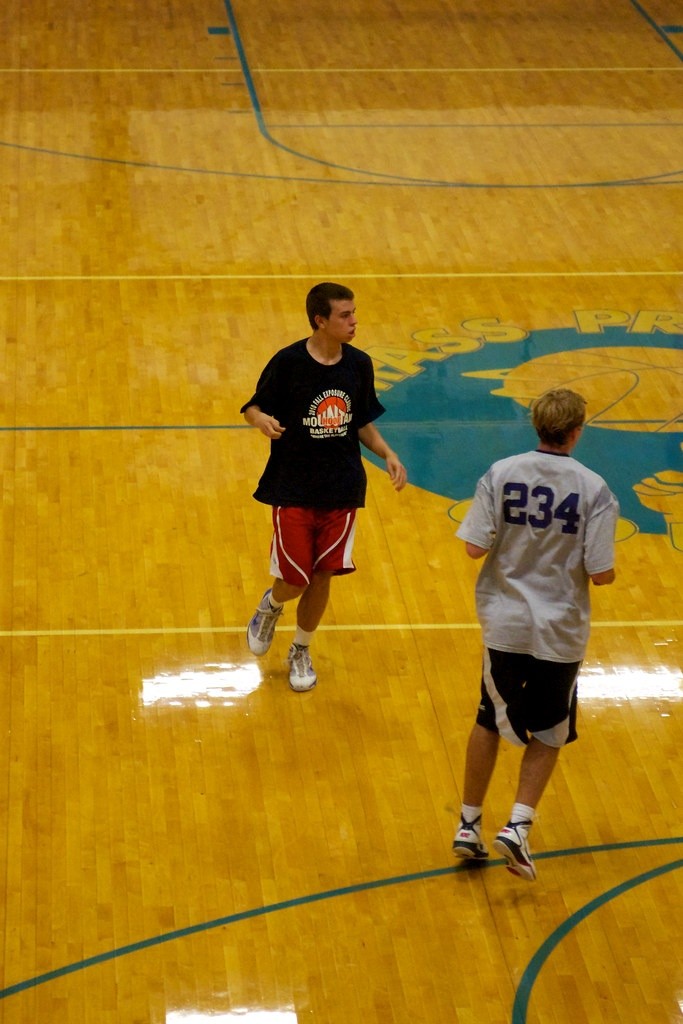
[
  {"xmin": 494, "ymin": 819, "xmax": 537, "ymax": 881},
  {"xmin": 288, "ymin": 642, "xmax": 318, "ymax": 691},
  {"xmin": 453, "ymin": 812, "xmax": 490, "ymax": 860},
  {"xmin": 247, "ymin": 588, "xmax": 284, "ymax": 657}
]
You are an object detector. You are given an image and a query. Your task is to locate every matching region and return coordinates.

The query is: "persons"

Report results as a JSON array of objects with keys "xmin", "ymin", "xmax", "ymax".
[
  {"xmin": 453, "ymin": 389, "xmax": 618, "ymax": 883},
  {"xmin": 240, "ymin": 282, "xmax": 407, "ymax": 692}
]
